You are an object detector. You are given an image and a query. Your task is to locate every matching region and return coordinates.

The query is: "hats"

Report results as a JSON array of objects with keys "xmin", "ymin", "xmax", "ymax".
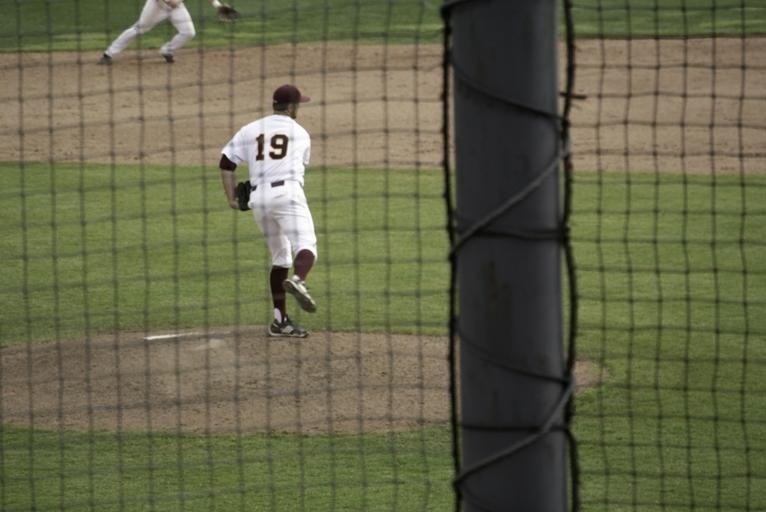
[{"xmin": 272, "ymin": 84, "xmax": 312, "ymax": 104}]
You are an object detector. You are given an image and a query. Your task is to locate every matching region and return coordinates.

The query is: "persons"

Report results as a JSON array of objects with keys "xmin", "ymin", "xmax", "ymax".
[
  {"xmin": 99, "ymin": 0, "xmax": 240, "ymax": 66},
  {"xmin": 219, "ymin": 85, "xmax": 319, "ymax": 338}
]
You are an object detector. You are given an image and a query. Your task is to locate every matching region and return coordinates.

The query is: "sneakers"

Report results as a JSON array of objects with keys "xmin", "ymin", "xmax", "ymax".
[
  {"xmin": 268, "ymin": 318, "xmax": 310, "ymax": 338},
  {"xmin": 99, "ymin": 53, "xmax": 113, "ymax": 65},
  {"xmin": 159, "ymin": 48, "xmax": 174, "ymax": 64},
  {"xmin": 281, "ymin": 274, "xmax": 318, "ymax": 314}
]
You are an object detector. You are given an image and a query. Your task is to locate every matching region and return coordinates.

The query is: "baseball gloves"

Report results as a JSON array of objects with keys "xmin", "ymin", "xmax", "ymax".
[
  {"xmin": 234, "ymin": 180, "xmax": 251, "ymax": 211},
  {"xmin": 217, "ymin": 4, "xmax": 241, "ymax": 22}
]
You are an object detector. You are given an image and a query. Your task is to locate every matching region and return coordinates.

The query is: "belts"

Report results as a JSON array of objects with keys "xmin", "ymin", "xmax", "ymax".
[{"xmin": 249, "ymin": 179, "xmax": 285, "ymax": 191}]
